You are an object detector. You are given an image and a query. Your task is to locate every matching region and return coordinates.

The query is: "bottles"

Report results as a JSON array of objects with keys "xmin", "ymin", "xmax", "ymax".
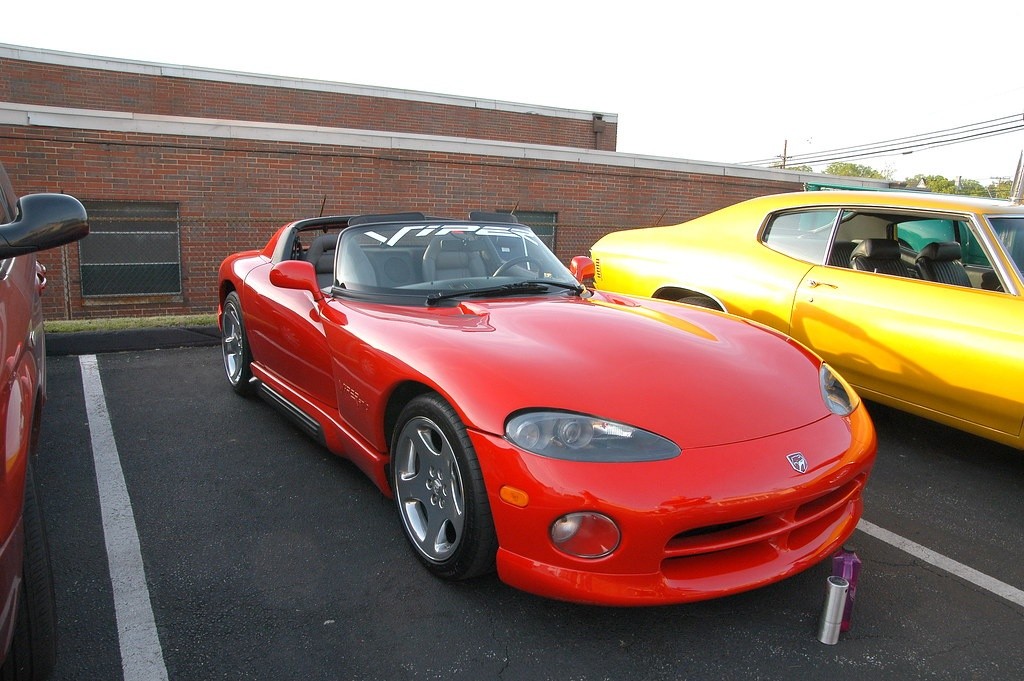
[{"xmin": 832, "ymin": 546, "xmax": 861, "ymax": 631}]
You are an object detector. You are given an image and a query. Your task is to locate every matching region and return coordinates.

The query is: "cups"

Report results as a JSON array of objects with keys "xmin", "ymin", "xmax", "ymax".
[{"xmin": 817, "ymin": 576, "xmax": 849, "ymax": 645}]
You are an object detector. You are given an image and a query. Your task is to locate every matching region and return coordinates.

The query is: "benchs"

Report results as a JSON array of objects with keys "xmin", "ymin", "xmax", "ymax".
[{"xmin": 787, "ymin": 240, "xmax": 858, "ymax": 268}]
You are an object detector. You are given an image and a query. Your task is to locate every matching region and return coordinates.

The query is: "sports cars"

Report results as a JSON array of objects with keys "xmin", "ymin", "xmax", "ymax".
[
  {"xmin": 1, "ymin": 157, "xmax": 91, "ymax": 681},
  {"xmin": 217, "ymin": 209, "xmax": 880, "ymax": 611},
  {"xmin": 589, "ymin": 190, "xmax": 1024, "ymax": 456}
]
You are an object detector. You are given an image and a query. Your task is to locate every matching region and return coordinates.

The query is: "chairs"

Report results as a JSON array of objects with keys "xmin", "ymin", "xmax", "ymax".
[
  {"xmin": 914, "ymin": 242, "xmax": 972, "ymax": 288},
  {"xmin": 422, "ymin": 235, "xmax": 486, "ymax": 281},
  {"xmin": 849, "ymin": 238, "xmax": 911, "ymax": 278},
  {"xmin": 306, "ymin": 235, "xmax": 377, "ymax": 287}
]
